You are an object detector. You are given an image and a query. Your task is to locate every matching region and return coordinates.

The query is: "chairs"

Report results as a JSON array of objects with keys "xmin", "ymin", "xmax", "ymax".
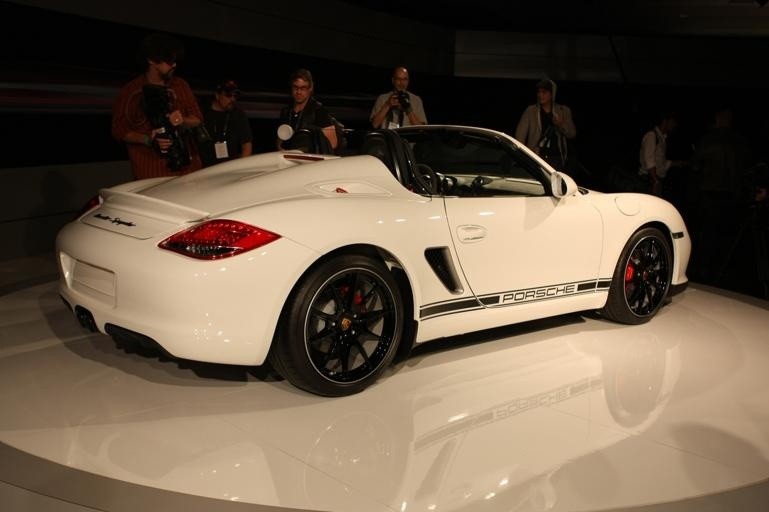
[{"xmin": 295, "ymin": 123, "xmax": 431, "ymax": 195}]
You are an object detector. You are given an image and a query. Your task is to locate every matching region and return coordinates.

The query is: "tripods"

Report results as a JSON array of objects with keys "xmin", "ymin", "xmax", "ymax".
[{"xmin": 720, "ymin": 177, "xmax": 769, "ymax": 299}]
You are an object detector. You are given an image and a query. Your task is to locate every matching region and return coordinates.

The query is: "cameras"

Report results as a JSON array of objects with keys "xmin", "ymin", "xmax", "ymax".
[{"xmin": 398, "ymin": 90, "xmax": 410, "ymax": 109}]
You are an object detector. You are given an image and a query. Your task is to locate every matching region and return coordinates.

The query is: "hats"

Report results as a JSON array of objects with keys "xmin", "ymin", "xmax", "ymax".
[{"xmin": 213, "ymin": 78, "xmax": 240, "ymax": 95}]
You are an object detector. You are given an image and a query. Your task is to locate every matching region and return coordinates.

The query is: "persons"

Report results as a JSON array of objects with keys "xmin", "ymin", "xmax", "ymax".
[
  {"xmin": 636, "ymin": 108, "xmax": 695, "ymax": 201},
  {"xmin": 369, "ymin": 65, "xmax": 430, "ymax": 150},
  {"xmin": 197, "ymin": 79, "xmax": 255, "ymax": 168},
  {"xmin": 274, "ymin": 64, "xmax": 340, "ymax": 154},
  {"xmin": 511, "ymin": 76, "xmax": 578, "ymax": 173},
  {"xmin": 111, "ymin": 34, "xmax": 206, "ymax": 185},
  {"xmin": 693, "ymin": 107, "xmax": 756, "ymax": 278}
]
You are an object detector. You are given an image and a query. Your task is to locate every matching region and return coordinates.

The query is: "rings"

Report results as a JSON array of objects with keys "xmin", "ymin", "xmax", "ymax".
[{"xmin": 175, "ymin": 118, "xmax": 179, "ymax": 123}]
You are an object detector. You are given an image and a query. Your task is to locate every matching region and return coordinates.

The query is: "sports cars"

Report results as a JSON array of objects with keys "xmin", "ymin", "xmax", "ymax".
[
  {"xmin": 52, "ymin": 122, "xmax": 698, "ymax": 397},
  {"xmin": 240, "ymin": 307, "xmax": 687, "ymax": 511}
]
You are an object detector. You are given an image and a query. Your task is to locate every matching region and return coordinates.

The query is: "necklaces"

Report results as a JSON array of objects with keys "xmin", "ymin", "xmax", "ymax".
[{"xmin": 294, "ymin": 104, "xmax": 301, "ymax": 118}]
